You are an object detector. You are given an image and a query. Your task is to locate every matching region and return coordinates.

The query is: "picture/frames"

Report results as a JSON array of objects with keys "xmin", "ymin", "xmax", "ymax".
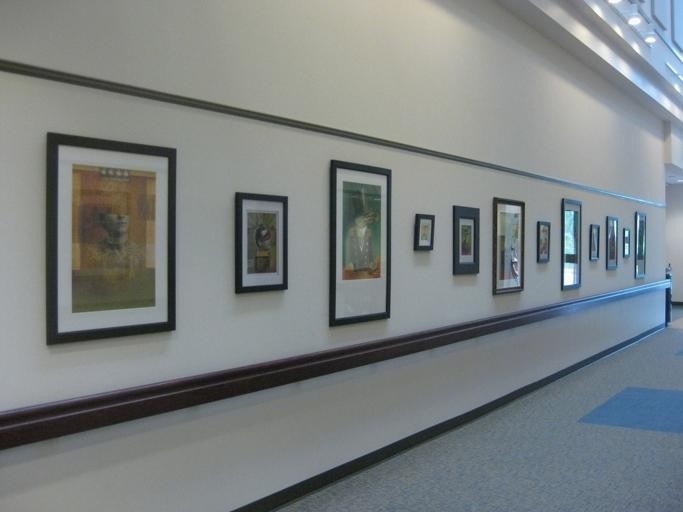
[
  {"xmin": 328, "ymin": 159, "xmax": 391, "ymax": 326},
  {"xmin": 233, "ymin": 191, "xmax": 287, "ymax": 295},
  {"xmin": 414, "ymin": 197, "xmax": 648, "ymax": 296},
  {"xmin": 44, "ymin": 128, "xmax": 178, "ymax": 348}
]
